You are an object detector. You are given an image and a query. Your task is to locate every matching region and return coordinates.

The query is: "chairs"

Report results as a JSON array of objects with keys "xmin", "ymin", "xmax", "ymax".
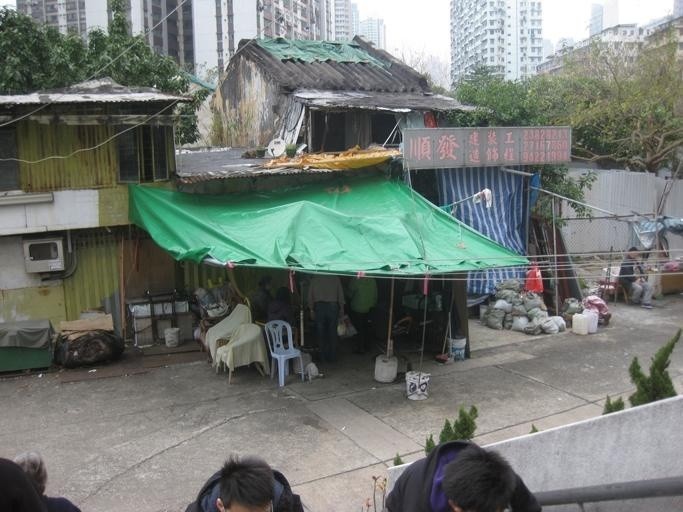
[
  {"xmin": 205, "ymin": 303, "xmax": 305, "ymax": 387},
  {"xmin": 614, "ymin": 276, "xmax": 633, "ymax": 307}
]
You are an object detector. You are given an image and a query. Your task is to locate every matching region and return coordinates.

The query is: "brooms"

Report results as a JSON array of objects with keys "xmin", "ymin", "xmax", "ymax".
[{"xmin": 434, "ymin": 286, "xmax": 456, "ymax": 362}]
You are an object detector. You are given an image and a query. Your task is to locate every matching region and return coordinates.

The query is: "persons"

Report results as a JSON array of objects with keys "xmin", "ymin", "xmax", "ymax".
[
  {"xmin": 12, "ymin": 453, "xmax": 83, "ymax": 512},
  {"xmin": 383, "ymin": 439, "xmax": 543, "ymax": 512},
  {"xmin": 251, "ymin": 272, "xmax": 379, "ymax": 362},
  {"xmin": 618, "ymin": 246, "xmax": 655, "ymax": 309},
  {"xmin": 184, "ymin": 452, "xmax": 303, "ymax": 512}
]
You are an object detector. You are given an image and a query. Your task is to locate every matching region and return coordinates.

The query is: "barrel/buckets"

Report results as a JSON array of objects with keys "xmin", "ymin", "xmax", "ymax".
[
  {"xmin": 583, "ymin": 308, "xmax": 599, "ymax": 333},
  {"xmin": 448, "ymin": 337, "xmax": 467, "ymax": 360},
  {"xmin": 164, "ymin": 327, "xmax": 180, "ymax": 348},
  {"xmin": 572, "ymin": 313, "xmax": 588, "ymax": 335}
]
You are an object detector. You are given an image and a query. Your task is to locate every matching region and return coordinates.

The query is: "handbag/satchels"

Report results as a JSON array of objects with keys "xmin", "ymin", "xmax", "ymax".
[{"xmin": 337, "ymin": 315, "xmax": 358, "ymax": 340}]
